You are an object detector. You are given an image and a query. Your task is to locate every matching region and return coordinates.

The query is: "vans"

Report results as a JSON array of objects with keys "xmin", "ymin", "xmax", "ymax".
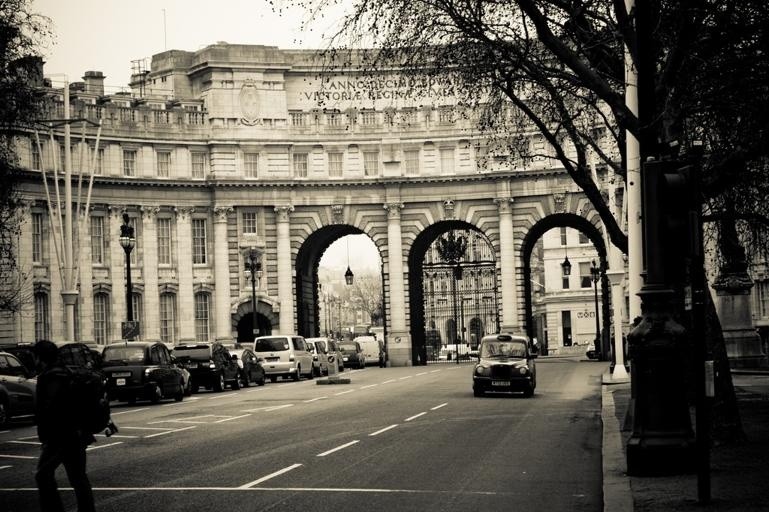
[{"xmin": 252, "ymin": 335, "xmax": 315, "ymax": 382}]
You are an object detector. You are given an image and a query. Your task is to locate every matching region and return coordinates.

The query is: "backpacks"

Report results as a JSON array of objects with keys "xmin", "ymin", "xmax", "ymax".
[{"xmin": 64, "ymin": 365, "xmax": 110, "ymax": 435}]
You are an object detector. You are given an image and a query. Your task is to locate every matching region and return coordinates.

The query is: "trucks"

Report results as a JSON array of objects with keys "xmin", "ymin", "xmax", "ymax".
[{"xmin": 369, "ymin": 327, "xmax": 385, "ymax": 345}]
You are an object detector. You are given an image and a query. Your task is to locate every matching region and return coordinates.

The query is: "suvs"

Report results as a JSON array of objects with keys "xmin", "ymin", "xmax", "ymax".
[
  {"xmin": 53, "ymin": 338, "xmax": 104, "ymax": 364},
  {"xmin": 169, "ymin": 341, "xmax": 241, "ymax": 394},
  {"xmin": 1, "ymin": 340, "xmax": 34, "ymax": 367}
]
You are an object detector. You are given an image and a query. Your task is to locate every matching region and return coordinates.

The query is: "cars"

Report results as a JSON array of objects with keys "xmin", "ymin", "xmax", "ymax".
[
  {"xmin": 0, "ymin": 351, "xmax": 38, "ymax": 429},
  {"xmin": 168, "ymin": 352, "xmax": 193, "ymax": 395},
  {"xmin": 471, "ymin": 334, "xmax": 538, "ymax": 397},
  {"xmin": 425, "ymin": 343, "xmax": 472, "ymax": 362},
  {"xmin": 100, "ymin": 342, "xmax": 186, "ymax": 403},
  {"xmin": 227, "ymin": 348, "xmax": 266, "ymax": 387},
  {"xmin": 302, "ymin": 334, "xmax": 385, "ymax": 374}
]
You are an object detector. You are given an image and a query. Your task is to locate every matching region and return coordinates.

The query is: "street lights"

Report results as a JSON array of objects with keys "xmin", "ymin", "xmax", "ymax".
[
  {"xmin": 590, "ymin": 259, "xmax": 602, "ymax": 361},
  {"xmin": 116, "ymin": 212, "xmax": 140, "ymax": 343},
  {"xmin": 242, "ymin": 244, "xmax": 263, "ymax": 339}
]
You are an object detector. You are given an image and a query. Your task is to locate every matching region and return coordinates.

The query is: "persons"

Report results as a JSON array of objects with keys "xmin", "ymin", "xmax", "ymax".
[{"xmin": 30, "ymin": 342, "xmax": 94, "ymax": 509}]
